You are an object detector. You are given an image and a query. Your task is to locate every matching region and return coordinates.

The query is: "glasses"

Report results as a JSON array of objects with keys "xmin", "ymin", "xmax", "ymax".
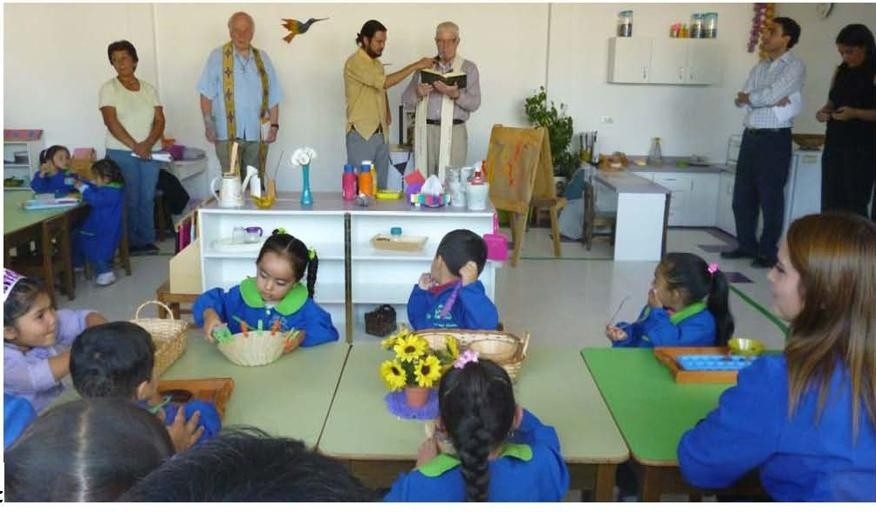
[{"xmin": 436, "ymin": 37, "xmax": 457, "ymax": 44}]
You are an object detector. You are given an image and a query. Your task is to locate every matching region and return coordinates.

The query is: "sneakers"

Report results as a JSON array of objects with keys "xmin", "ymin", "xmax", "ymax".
[
  {"xmin": 129, "ymin": 246, "xmax": 141, "ymax": 255},
  {"xmin": 142, "ymin": 243, "xmax": 160, "ymax": 254},
  {"xmin": 95, "ymin": 270, "xmax": 116, "ymax": 286}
]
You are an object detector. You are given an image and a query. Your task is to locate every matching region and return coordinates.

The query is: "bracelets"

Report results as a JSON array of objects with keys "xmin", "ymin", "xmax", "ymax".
[
  {"xmin": 270, "ymin": 123, "xmax": 279, "ymax": 129},
  {"xmin": 447, "ymin": 91, "xmax": 461, "ymax": 100}
]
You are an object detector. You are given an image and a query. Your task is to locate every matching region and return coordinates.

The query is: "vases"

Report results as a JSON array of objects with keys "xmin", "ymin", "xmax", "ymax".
[
  {"xmin": 300, "ymin": 166, "xmax": 313, "ymax": 207},
  {"xmin": 405, "ymin": 384, "xmax": 430, "ymax": 409}
]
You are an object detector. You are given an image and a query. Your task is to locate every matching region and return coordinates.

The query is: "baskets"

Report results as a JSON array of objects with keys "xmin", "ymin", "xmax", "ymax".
[
  {"xmin": 217, "ymin": 329, "xmax": 287, "ymax": 366},
  {"xmin": 370, "ymin": 231, "xmax": 427, "ymax": 250},
  {"xmin": 364, "ymin": 304, "xmax": 397, "ymax": 337},
  {"xmin": 128, "ymin": 300, "xmax": 188, "ymax": 382},
  {"xmin": 410, "ymin": 328, "xmax": 530, "ymax": 386}
]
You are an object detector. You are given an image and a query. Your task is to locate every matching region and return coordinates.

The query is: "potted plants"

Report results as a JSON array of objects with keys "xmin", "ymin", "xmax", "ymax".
[{"xmin": 524, "ymin": 87, "xmax": 576, "ymax": 196}]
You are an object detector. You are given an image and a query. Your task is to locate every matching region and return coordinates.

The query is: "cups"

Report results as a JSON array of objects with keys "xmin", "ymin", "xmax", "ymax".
[
  {"xmin": 468, "ymin": 185, "xmax": 486, "ymax": 209},
  {"xmin": 450, "ymin": 185, "xmax": 465, "ymax": 208}
]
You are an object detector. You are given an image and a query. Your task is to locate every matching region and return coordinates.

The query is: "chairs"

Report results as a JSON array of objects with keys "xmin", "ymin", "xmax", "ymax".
[
  {"xmin": 582, "ymin": 181, "xmax": 617, "ymax": 250},
  {"xmin": 11, "ymin": 212, "xmax": 74, "ymax": 308},
  {"xmin": 156, "ymin": 277, "xmax": 199, "ymax": 320},
  {"xmin": 84, "ymin": 201, "xmax": 131, "ymax": 282}
]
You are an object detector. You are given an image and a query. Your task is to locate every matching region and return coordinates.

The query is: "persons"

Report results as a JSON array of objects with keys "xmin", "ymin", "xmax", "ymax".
[
  {"xmin": 75, "ymin": 159, "xmax": 126, "ymax": 287},
  {"xmin": 719, "ymin": 16, "xmax": 807, "ymax": 269},
  {"xmin": 71, "ymin": 322, "xmax": 217, "ymax": 447},
  {"xmin": 95, "ymin": 40, "xmax": 165, "ymax": 253},
  {"xmin": 383, "ymin": 350, "xmax": 570, "ymax": 503},
  {"xmin": 408, "ymin": 230, "xmax": 502, "ymax": 334},
  {"xmin": 4, "ymin": 266, "xmax": 110, "ymax": 412},
  {"xmin": 580, "ymin": 252, "xmax": 736, "ymax": 502},
  {"xmin": 673, "ymin": 213, "xmax": 876, "ymax": 503},
  {"xmin": 122, "ymin": 426, "xmax": 371, "ymax": 503},
  {"xmin": 343, "ymin": 20, "xmax": 438, "ymax": 191},
  {"xmin": 815, "ymin": 22, "xmax": 876, "ymax": 217},
  {"xmin": 402, "ymin": 21, "xmax": 482, "ymax": 182},
  {"xmin": 195, "ymin": 12, "xmax": 285, "ymax": 189},
  {"xmin": 29, "ymin": 143, "xmax": 86, "ymax": 263},
  {"xmin": 194, "ymin": 229, "xmax": 340, "ymax": 352},
  {"xmin": 2, "ymin": 401, "xmax": 205, "ymax": 501}
]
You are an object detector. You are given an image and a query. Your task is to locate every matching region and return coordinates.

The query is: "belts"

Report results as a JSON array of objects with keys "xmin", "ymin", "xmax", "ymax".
[
  {"xmin": 750, "ymin": 128, "xmax": 788, "ymax": 134},
  {"xmin": 376, "ymin": 128, "xmax": 382, "ymax": 133},
  {"xmin": 428, "ymin": 120, "xmax": 463, "ymax": 124}
]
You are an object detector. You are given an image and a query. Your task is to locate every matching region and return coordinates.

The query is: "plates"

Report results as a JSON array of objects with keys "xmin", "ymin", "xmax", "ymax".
[
  {"xmin": 653, "ymin": 346, "xmax": 737, "ymax": 382},
  {"xmin": 157, "ymin": 378, "xmax": 234, "ymax": 418},
  {"xmin": 371, "ymin": 233, "xmax": 428, "ymax": 252},
  {"xmin": 213, "ymin": 236, "xmax": 264, "ymax": 250}
]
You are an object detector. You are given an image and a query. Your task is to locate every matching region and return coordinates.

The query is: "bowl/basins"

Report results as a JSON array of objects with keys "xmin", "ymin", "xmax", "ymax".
[
  {"xmin": 729, "ymin": 338, "xmax": 763, "ymax": 355},
  {"xmin": 469, "ymin": 339, "xmax": 518, "ymax": 362}
]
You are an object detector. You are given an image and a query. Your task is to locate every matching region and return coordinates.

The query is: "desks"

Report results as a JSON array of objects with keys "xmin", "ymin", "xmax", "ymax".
[
  {"xmin": 4, "ymin": 190, "xmax": 87, "ymax": 295},
  {"xmin": 37, "ymin": 341, "xmax": 351, "ymax": 453},
  {"xmin": 581, "ymin": 346, "xmax": 778, "ymax": 501},
  {"xmin": 317, "ymin": 344, "xmax": 630, "ymax": 501}
]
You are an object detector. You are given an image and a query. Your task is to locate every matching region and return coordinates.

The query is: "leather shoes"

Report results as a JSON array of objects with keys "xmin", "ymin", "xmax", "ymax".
[
  {"xmin": 751, "ymin": 253, "xmax": 777, "ymax": 268},
  {"xmin": 721, "ymin": 248, "xmax": 756, "ymax": 259}
]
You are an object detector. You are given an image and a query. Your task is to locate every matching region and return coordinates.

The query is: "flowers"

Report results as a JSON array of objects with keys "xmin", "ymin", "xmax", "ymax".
[
  {"xmin": 380, "ymin": 323, "xmax": 479, "ymax": 393},
  {"xmin": 291, "ymin": 148, "xmax": 317, "ymax": 167}
]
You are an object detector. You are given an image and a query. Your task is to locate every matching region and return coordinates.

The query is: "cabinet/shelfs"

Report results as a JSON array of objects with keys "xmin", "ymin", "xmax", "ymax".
[
  {"xmin": 782, "ymin": 151, "xmax": 822, "ymax": 237},
  {"xmin": 719, "ymin": 173, "xmax": 737, "ymax": 239},
  {"xmin": 653, "ymin": 172, "xmax": 684, "ymax": 227},
  {"xmin": 684, "ymin": 174, "xmax": 720, "ymax": 231},
  {"xmin": 198, "ymin": 192, "xmax": 496, "ymax": 329},
  {"xmin": 4, "ymin": 129, "xmax": 45, "ymax": 188},
  {"xmin": 607, "ymin": 37, "xmax": 717, "ymax": 85}
]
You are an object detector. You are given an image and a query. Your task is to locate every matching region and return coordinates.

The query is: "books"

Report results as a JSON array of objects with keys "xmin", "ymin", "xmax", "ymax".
[{"xmin": 420, "ymin": 68, "xmax": 468, "ymax": 89}]
